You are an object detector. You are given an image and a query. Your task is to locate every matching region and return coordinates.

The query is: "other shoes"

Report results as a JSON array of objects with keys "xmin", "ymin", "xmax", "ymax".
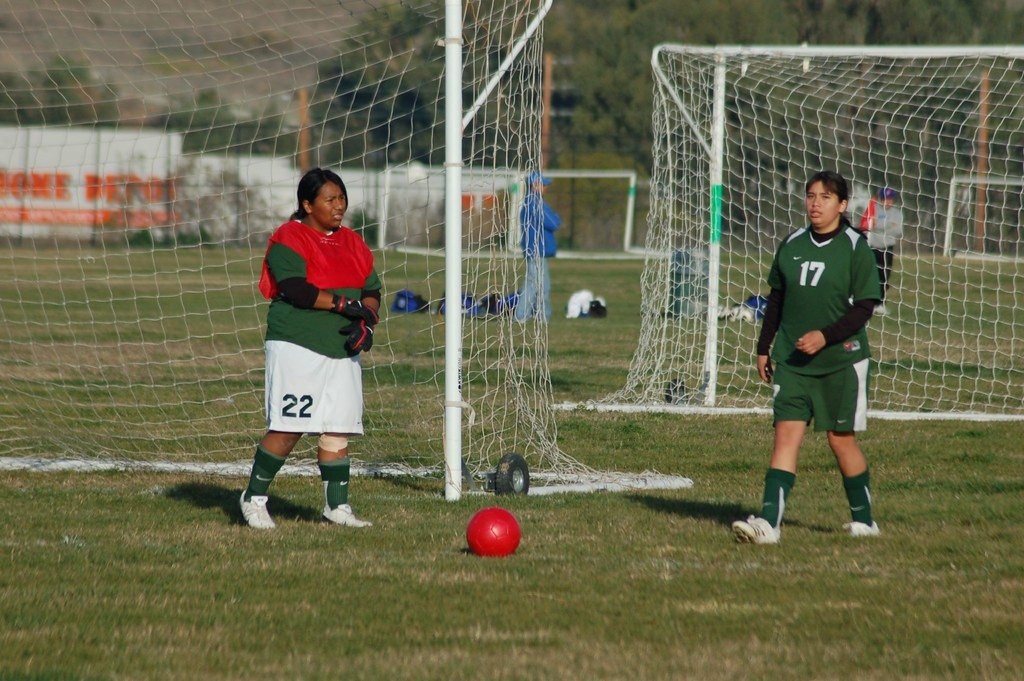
[{"xmin": 873, "ymin": 305, "xmax": 889, "ymax": 315}]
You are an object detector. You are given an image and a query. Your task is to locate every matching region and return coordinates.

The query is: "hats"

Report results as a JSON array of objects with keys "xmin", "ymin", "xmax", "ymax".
[
  {"xmin": 525, "ymin": 171, "xmax": 551, "ymax": 185},
  {"xmin": 878, "ymin": 188, "xmax": 895, "ymax": 200}
]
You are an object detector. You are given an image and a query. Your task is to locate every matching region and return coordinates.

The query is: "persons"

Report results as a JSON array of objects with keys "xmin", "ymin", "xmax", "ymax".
[
  {"xmin": 732, "ymin": 171, "xmax": 883, "ymax": 546},
  {"xmin": 512, "ymin": 170, "xmax": 562, "ymax": 326},
  {"xmin": 238, "ymin": 168, "xmax": 384, "ymax": 533},
  {"xmin": 856, "ymin": 189, "xmax": 905, "ymax": 316}
]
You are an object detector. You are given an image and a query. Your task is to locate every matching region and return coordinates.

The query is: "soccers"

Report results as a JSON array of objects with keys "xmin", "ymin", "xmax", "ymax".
[{"xmin": 466, "ymin": 507, "xmax": 522, "ymax": 557}]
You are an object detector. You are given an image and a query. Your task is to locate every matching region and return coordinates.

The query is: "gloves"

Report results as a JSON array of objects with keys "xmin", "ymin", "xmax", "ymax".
[
  {"xmin": 338, "ymin": 317, "xmax": 373, "ymax": 356},
  {"xmin": 331, "ymin": 294, "xmax": 380, "ymax": 327}
]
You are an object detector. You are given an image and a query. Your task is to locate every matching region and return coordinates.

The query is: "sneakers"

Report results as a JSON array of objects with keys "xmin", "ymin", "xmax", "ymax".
[
  {"xmin": 240, "ymin": 490, "xmax": 275, "ymax": 529},
  {"xmin": 848, "ymin": 520, "xmax": 880, "ymax": 539},
  {"xmin": 732, "ymin": 516, "xmax": 780, "ymax": 544},
  {"xmin": 321, "ymin": 504, "xmax": 372, "ymax": 528}
]
